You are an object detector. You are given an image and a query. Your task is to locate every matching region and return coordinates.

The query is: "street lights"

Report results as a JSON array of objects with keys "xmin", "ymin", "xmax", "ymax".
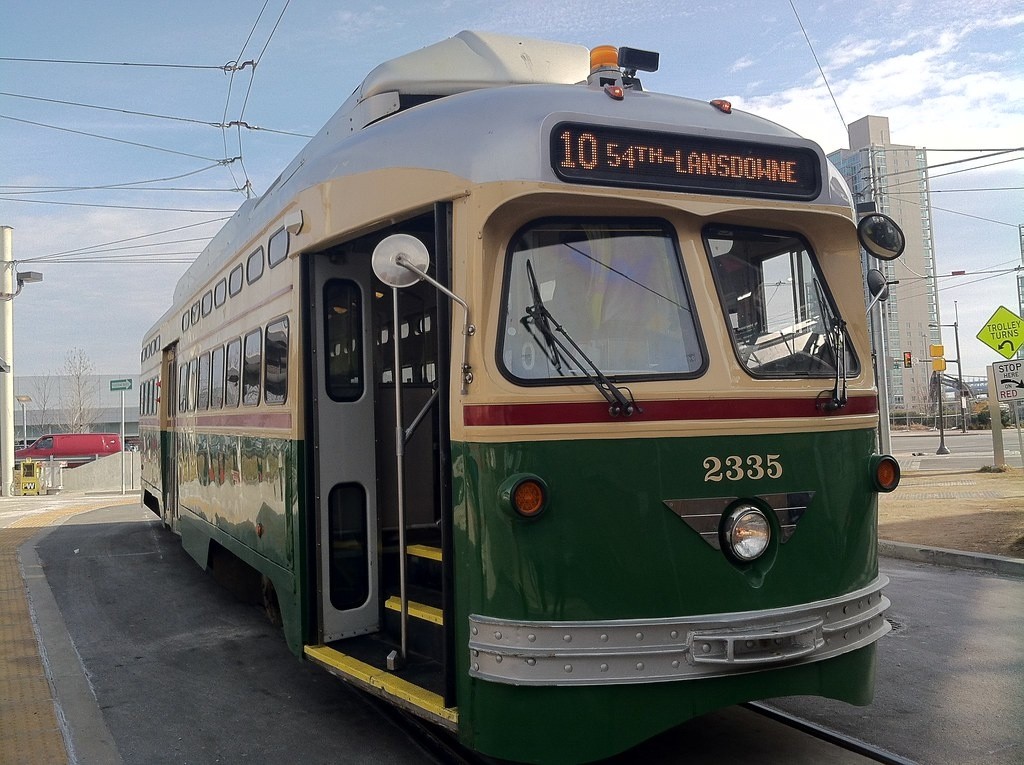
[{"xmin": 927, "ymin": 321, "xmax": 969, "ymax": 433}]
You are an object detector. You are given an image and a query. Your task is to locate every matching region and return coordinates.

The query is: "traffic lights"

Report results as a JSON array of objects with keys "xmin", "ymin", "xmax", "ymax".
[{"xmin": 903, "ymin": 351, "xmax": 913, "ymax": 369}]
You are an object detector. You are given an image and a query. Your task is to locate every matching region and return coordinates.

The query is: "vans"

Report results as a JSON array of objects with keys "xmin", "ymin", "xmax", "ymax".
[{"xmin": 14, "ymin": 432, "xmax": 123, "ymax": 458}]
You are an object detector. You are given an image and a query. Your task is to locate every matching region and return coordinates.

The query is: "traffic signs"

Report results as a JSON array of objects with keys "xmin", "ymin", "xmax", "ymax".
[{"xmin": 109, "ymin": 378, "xmax": 132, "ymax": 391}]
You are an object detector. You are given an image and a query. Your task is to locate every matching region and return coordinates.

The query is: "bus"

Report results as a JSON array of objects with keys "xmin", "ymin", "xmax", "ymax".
[{"xmin": 138, "ymin": 29, "xmax": 907, "ymax": 765}]
